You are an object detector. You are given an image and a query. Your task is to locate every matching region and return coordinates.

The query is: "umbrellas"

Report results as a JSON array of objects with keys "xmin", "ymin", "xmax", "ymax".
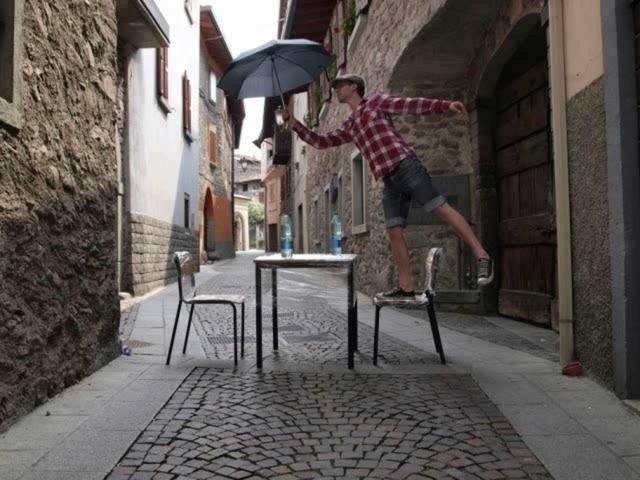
[{"xmin": 216, "ymin": 38, "xmax": 337, "ymax": 123}]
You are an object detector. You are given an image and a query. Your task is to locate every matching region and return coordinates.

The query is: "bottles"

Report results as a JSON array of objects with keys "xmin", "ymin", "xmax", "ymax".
[
  {"xmin": 328, "ymin": 211, "xmax": 344, "ymax": 256},
  {"xmin": 278, "ymin": 213, "xmax": 294, "ymax": 259}
]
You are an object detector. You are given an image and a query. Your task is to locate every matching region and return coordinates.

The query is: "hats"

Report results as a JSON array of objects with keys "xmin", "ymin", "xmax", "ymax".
[{"xmin": 328, "ymin": 74, "xmax": 365, "ymax": 88}]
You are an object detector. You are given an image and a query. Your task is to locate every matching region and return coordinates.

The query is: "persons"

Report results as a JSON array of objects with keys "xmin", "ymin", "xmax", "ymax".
[{"xmin": 281, "ymin": 72, "xmax": 495, "ymax": 301}]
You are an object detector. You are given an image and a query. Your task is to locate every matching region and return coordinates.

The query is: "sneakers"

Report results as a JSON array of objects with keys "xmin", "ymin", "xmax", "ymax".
[
  {"xmin": 476, "ymin": 257, "xmax": 495, "ymax": 286},
  {"xmin": 375, "ymin": 286, "xmax": 415, "ymax": 300}
]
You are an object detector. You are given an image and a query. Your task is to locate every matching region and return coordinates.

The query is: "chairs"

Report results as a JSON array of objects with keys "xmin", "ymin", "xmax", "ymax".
[{"xmin": 166, "ymin": 247, "xmax": 452, "ymax": 371}]
